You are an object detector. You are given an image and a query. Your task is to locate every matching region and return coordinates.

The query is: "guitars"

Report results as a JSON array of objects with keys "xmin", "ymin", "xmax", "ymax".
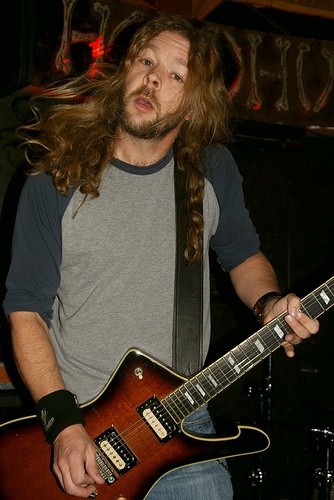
[{"xmin": 0, "ymin": 275, "xmax": 334, "ymax": 500}]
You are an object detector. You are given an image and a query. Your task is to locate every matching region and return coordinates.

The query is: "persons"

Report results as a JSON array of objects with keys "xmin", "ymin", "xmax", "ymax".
[{"xmin": 4, "ymin": 14, "xmax": 319, "ymax": 497}]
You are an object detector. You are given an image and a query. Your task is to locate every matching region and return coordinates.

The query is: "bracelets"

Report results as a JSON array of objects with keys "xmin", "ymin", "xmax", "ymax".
[
  {"xmin": 34, "ymin": 389, "xmax": 85, "ymax": 443},
  {"xmin": 252, "ymin": 292, "xmax": 281, "ymax": 324}
]
